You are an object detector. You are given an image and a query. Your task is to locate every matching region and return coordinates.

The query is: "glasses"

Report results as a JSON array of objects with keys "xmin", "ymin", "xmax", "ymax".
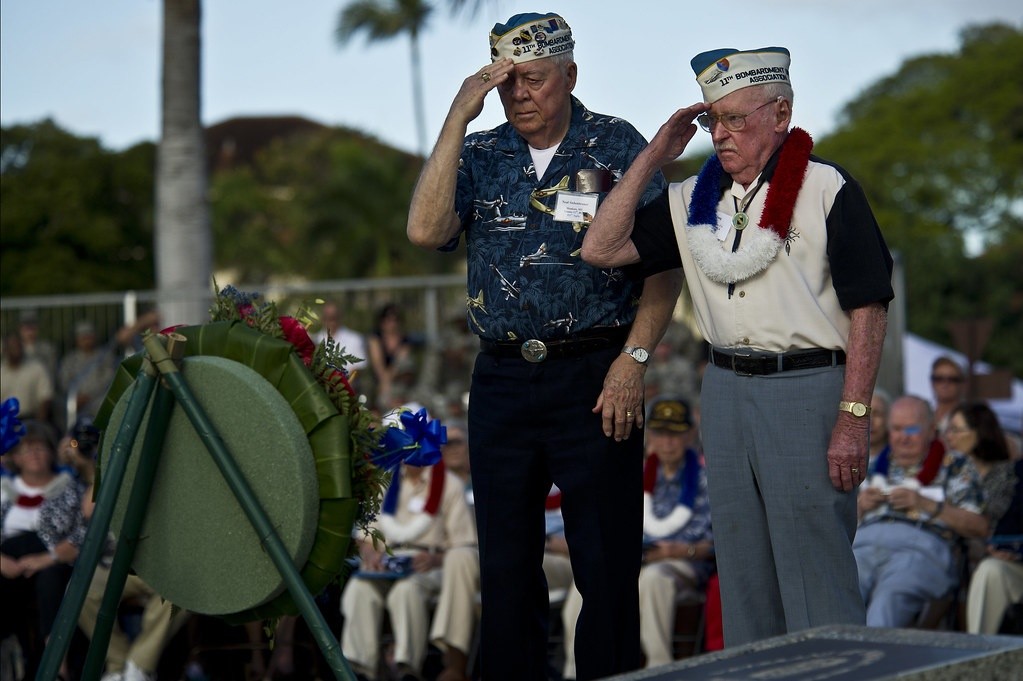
[
  {"xmin": 931, "ymin": 375, "xmax": 962, "ymax": 385},
  {"xmin": 697, "ymin": 99, "xmax": 778, "ymax": 133}
]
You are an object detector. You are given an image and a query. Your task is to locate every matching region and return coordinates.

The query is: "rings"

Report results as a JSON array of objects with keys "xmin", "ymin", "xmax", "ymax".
[
  {"xmin": 627, "ymin": 411, "xmax": 634, "ymax": 417},
  {"xmin": 852, "ymin": 467, "xmax": 859, "ymax": 473},
  {"xmin": 481, "ymin": 73, "xmax": 491, "ymax": 82}
]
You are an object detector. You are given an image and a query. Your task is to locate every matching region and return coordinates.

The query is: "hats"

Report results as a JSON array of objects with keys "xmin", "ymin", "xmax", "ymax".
[
  {"xmin": 646, "ymin": 394, "xmax": 694, "ymax": 432},
  {"xmin": 690, "ymin": 47, "xmax": 792, "ymax": 105},
  {"xmin": 489, "ymin": 12, "xmax": 576, "ymax": 65}
]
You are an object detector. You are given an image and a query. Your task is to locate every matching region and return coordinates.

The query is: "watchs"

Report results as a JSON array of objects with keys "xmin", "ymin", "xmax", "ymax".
[
  {"xmin": 839, "ymin": 400, "xmax": 872, "ymax": 418},
  {"xmin": 621, "ymin": 345, "xmax": 651, "ymax": 368},
  {"xmin": 686, "ymin": 543, "xmax": 696, "ymax": 560}
]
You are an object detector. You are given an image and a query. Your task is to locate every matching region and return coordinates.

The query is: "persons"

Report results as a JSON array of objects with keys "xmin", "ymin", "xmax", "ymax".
[
  {"xmin": 580, "ymin": 45, "xmax": 895, "ymax": 647},
  {"xmin": 407, "ymin": 12, "xmax": 685, "ymax": 681},
  {"xmin": 0, "ymin": 313, "xmax": 187, "ymax": 681},
  {"xmin": 852, "ymin": 358, "xmax": 1023, "ymax": 639},
  {"xmin": 239, "ymin": 296, "xmax": 716, "ymax": 681}
]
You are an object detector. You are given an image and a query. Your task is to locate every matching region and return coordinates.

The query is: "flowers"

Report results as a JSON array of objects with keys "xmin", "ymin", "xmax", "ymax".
[{"xmin": 91, "ymin": 274, "xmax": 452, "ymax": 650}]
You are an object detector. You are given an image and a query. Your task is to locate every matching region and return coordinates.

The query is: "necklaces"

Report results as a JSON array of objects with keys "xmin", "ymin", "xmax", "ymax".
[
  {"xmin": 686, "ymin": 127, "xmax": 814, "ymax": 283},
  {"xmin": 378, "ymin": 458, "xmax": 445, "ymax": 543},
  {"xmin": 723, "ymin": 181, "xmax": 765, "ymax": 299},
  {"xmin": 2, "ymin": 464, "xmax": 76, "ymax": 508},
  {"xmin": 869, "ymin": 438, "xmax": 948, "ymax": 502},
  {"xmin": 642, "ymin": 448, "xmax": 699, "ymax": 538},
  {"xmin": 546, "ymin": 491, "xmax": 560, "ymax": 511}
]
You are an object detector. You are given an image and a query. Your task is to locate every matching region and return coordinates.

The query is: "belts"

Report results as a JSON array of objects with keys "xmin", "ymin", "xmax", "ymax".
[
  {"xmin": 480, "ymin": 324, "xmax": 632, "ymax": 362},
  {"xmin": 709, "ymin": 349, "xmax": 844, "ymax": 378},
  {"xmin": 858, "ymin": 515, "xmax": 948, "ymax": 543}
]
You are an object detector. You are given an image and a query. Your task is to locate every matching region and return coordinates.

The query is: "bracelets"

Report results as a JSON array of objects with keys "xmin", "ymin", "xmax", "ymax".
[
  {"xmin": 48, "ymin": 552, "xmax": 61, "ymax": 565},
  {"xmin": 930, "ymin": 502, "xmax": 943, "ymax": 521}
]
